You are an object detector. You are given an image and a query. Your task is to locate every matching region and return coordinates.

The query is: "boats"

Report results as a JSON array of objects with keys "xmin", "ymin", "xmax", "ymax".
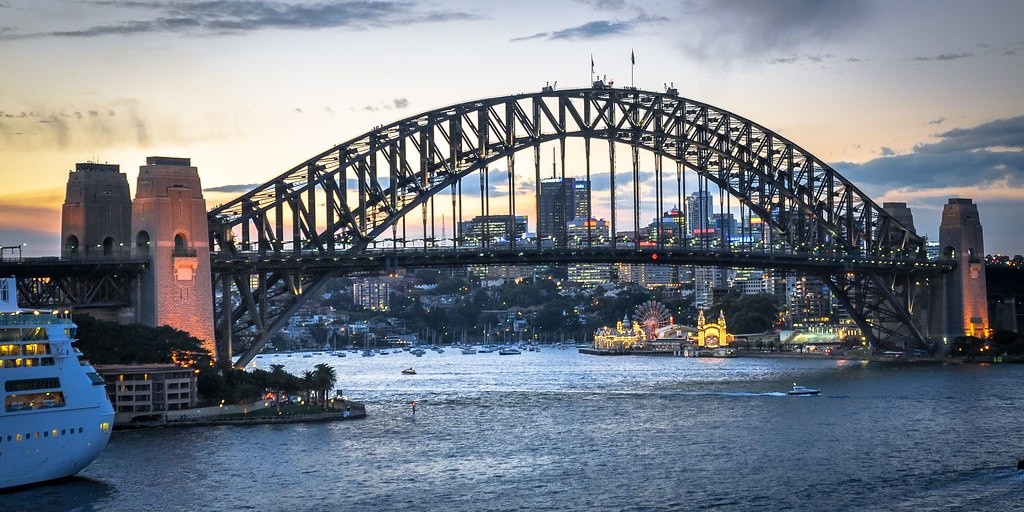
[
  {"xmin": 712, "ymin": 348, "xmax": 736, "ymax": 357},
  {"xmin": 0, "ymin": 274, "xmax": 115, "ymax": 491},
  {"xmin": 788, "ymin": 383, "xmax": 821, "ymax": 397},
  {"xmin": 402, "ymin": 366, "xmax": 417, "ymax": 375}
]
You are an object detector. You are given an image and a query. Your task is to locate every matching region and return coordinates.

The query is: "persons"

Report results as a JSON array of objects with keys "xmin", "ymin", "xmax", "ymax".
[
  {"xmin": 663, "ymin": 81, "xmax": 674, "ymax": 92},
  {"xmin": 546, "ymin": 81, "xmax": 557, "ymax": 90},
  {"xmin": 597, "ymin": 75, "xmax": 613, "ymax": 88}
]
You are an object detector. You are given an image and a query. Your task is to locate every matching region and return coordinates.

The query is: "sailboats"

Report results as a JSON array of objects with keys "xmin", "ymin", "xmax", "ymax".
[{"xmin": 254, "ymin": 322, "xmax": 592, "ymax": 356}]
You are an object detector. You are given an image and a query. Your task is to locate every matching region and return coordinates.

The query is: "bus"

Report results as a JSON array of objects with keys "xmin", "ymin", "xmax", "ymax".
[
  {"xmin": 884, "ymin": 351, "xmax": 906, "ymax": 359},
  {"xmin": 906, "ymin": 348, "xmax": 927, "ymax": 358}
]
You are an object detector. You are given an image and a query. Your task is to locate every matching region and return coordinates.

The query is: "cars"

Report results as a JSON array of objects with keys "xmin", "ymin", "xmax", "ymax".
[{"xmin": 948, "ymin": 336, "xmax": 985, "ymax": 358}]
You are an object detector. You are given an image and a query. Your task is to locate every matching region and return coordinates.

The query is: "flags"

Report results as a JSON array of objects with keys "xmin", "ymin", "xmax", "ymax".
[
  {"xmin": 631, "ymin": 50, "xmax": 635, "ymax": 65},
  {"xmin": 592, "ymin": 58, "xmax": 594, "ymax": 73}
]
